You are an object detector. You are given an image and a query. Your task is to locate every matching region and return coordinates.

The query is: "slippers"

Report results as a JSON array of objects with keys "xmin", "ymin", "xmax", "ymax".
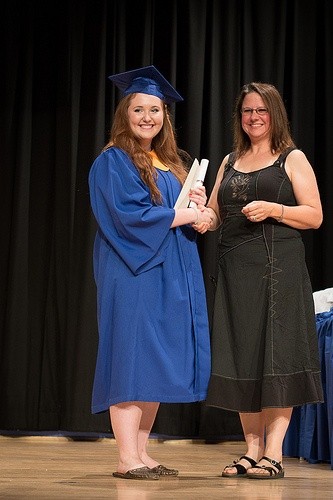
[
  {"xmin": 112, "ymin": 464, "xmax": 180, "ymax": 480},
  {"xmin": 221, "ymin": 455, "xmax": 285, "ymax": 479}
]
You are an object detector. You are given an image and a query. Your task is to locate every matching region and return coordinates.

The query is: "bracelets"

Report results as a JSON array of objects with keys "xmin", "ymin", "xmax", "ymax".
[
  {"xmin": 277, "ymin": 203, "xmax": 284, "ymax": 222},
  {"xmin": 192, "ymin": 82, "xmax": 325, "ymax": 480},
  {"xmin": 194, "ymin": 208, "xmax": 199, "ymax": 226}
]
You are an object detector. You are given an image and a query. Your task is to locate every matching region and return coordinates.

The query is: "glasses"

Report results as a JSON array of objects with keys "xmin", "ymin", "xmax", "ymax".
[{"xmin": 241, "ymin": 107, "xmax": 268, "ymax": 115}]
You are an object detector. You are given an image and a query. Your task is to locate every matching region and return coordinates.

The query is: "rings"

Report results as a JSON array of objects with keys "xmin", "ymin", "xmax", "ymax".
[{"xmin": 253, "ymin": 215, "xmax": 257, "ymax": 222}]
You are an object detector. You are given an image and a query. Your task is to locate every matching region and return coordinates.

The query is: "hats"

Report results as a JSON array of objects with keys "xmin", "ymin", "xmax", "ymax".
[{"xmin": 108, "ymin": 65, "xmax": 185, "ymax": 104}]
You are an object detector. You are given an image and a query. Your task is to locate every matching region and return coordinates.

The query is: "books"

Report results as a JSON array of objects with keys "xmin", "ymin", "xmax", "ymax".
[{"xmin": 173, "ymin": 157, "xmax": 210, "ymax": 210}]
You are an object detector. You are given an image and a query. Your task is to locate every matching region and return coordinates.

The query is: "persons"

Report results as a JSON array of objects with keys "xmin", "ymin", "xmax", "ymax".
[{"xmin": 88, "ymin": 91, "xmax": 217, "ymax": 481}]
